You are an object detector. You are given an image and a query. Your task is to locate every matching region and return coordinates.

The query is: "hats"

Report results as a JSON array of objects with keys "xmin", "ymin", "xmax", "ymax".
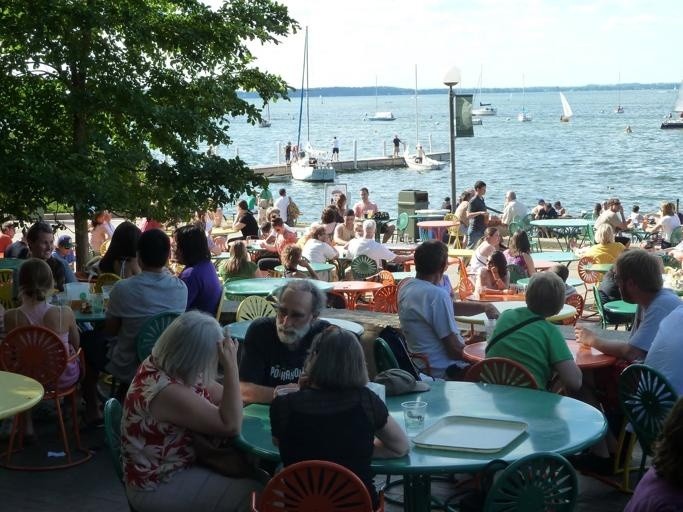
[
  {"xmin": 266, "ymin": 207, "xmax": 279, "ymax": 223},
  {"xmin": 58, "ymin": 234, "xmax": 76, "ymax": 249},
  {"xmin": 373, "ymin": 369, "xmax": 430, "ymax": 394},
  {"xmin": 344, "ymin": 209, "xmax": 354, "ymax": 216}
]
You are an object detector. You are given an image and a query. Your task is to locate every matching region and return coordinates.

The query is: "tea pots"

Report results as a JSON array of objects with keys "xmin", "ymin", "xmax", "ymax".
[{"xmin": 63, "ymin": 282, "xmax": 90, "ymax": 309}]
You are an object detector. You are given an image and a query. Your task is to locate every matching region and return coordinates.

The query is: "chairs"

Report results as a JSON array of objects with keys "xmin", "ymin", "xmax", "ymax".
[
  {"xmin": 372, "ymin": 285, "xmax": 398, "ymax": 314},
  {"xmin": 373, "ymin": 338, "xmax": 399, "ymax": 373},
  {"xmin": 662, "ymin": 255, "xmax": 681, "ymax": 269},
  {"xmin": 577, "ymin": 256, "xmax": 600, "ymax": 319},
  {"xmin": 0, "ymin": 269, "xmax": 22, "ymax": 310},
  {"xmin": 513, "ymin": 215, "xmax": 528, "ymax": 230},
  {"xmin": 351, "ymin": 254, "xmax": 377, "ymax": 281},
  {"xmin": 443, "ymin": 213, "xmax": 463, "ymax": 249},
  {"xmin": 508, "ymin": 222, "xmax": 538, "ymax": 253},
  {"xmin": 104, "ymin": 398, "xmax": 136, "ymax": 512},
  {"xmin": 325, "ymin": 291, "xmax": 347, "ymax": 310},
  {"xmin": 458, "ymin": 278, "xmax": 475, "ymax": 302},
  {"xmin": 507, "ymin": 265, "xmax": 526, "ymax": 284},
  {"xmin": 97, "ymin": 273, "xmax": 122, "ymax": 286},
  {"xmin": 586, "ymin": 223, "xmax": 597, "ymax": 246},
  {"xmin": 670, "ymin": 225, "xmax": 683, "ymax": 247},
  {"xmin": 216, "ymin": 287, "xmax": 227, "ymax": 321},
  {"xmin": 0, "ymin": 325, "xmax": 93, "ymax": 470},
  {"xmin": 136, "ymin": 311, "xmax": 183, "ymax": 363},
  {"xmin": 464, "ymin": 357, "xmax": 540, "ymax": 389},
  {"xmin": 618, "ymin": 364, "xmax": 679, "ymax": 461},
  {"xmin": 377, "ymin": 269, "xmax": 395, "ymax": 285},
  {"xmin": 487, "ymin": 452, "xmax": 580, "ymax": 512},
  {"xmin": 236, "ymin": 296, "xmax": 276, "ymax": 322},
  {"xmin": 523, "ymin": 214, "xmax": 535, "ymax": 230},
  {"xmin": 388, "ymin": 212, "xmax": 409, "ymax": 243},
  {"xmin": 458, "ymin": 258, "xmax": 471, "ymax": 281},
  {"xmin": 593, "ymin": 285, "xmax": 630, "ymax": 332},
  {"xmin": 248, "ymin": 461, "xmax": 384, "ymax": 512},
  {"xmin": 556, "ymin": 294, "xmax": 584, "ymax": 325}
]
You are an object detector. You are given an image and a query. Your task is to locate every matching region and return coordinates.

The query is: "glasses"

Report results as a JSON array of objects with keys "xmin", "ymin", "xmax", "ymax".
[
  {"xmin": 611, "ymin": 202, "xmax": 620, "ymax": 206},
  {"xmin": 278, "ymin": 306, "xmax": 305, "ymax": 318},
  {"xmin": 613, "ymin": 275, "xmax": 620, "ymax": 281}
]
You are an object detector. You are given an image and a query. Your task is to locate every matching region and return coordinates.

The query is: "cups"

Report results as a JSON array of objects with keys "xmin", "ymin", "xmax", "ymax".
[
  {"xmin": 577, "ymin": 323, "xmax": 595, "ymax": 353},
  {"xmin": 402, "ymin": 402, "xmax": 427, "ymax": 437},
  {"xmin": 485, "ymin": 318, "xmax": 496, "ymax": 342},
  {"xmin": 92, "ymin": 294, "xmax": 104, "ymax": 314},
  {"xmin": 102, "ymin": 286, "xmax": 112, "ymax": 297},
  {"xmin": 61, "ymin": 295, "xmax": 71, "ymax": 307},
  {"xmin": 247, "ymin": 236, "xmax": 251, "ymax": 249},
  {"xmin": 278, "ymin": 387, "xmax": 298, "ymax": 396}
]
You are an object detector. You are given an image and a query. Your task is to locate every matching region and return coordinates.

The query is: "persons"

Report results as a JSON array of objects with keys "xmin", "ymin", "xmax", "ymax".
[
  {"xmin": 95, "ymin": 221, "xmax": 142, "ymax": 291},
  {"xmin": 574, "ymin": 251, "xmax": 683, "ymax": 465},
  {"xmin": 536, "ymin": 203, "xmax": 558, "ymax": 222},
  {"xmin": 531, "ymin": 200, "xmax": 544, "ymax": 216},
  {"xmin": 90, "ymin": 210, "xmax": 105, "ymax": 247},
  {"xmin": 502, "ymin": 191, "xmax": 527, "ymax": 224},
  {"xmin": 219, "ymin": 241, "xmax": 262, "ymax": 301},
  {"xmin": 318, "ymin": 208, "xmax": 338, "ymax": 241},
  {"xmin": 417, "ymin": 146, "xmax": 425, "ymax": 163},
  {"xmin": 353, "ymin": 187, "xmax": 395, "ymax": 243},
  {"xmin": 554, "ymin": 201, "xmax": 564, "ymax": 215},
  {"xmin": 393, "ymin": 135, "xmax": 403, "ymax": 158},
  {"xmin": 469, "ymin": 181, "xmax": 488, "ymax": 248},
  {"xmin": 503, "ymin": 231, "xmax": 535, "ymax": 280},
  {"xmin": 173, "ymin": 225, "xmax": 222, "ymax": 314},
  {"xmin": 454, "ymin": 192, "xmax": 469, "ymax": 249},
  {"xmin": 121, "ymin": 310, "xmax": 263, "ymax": 511},
  {"xmin": 27, "ymin": 221, "xmax": 78, "ymax": 284},
  {"xmin": 485, "ymin": 271, "xmax": 583, "ymax": 390},
  {"xmin": 82, "ymin": 229, "xmax": 188, "ymax": 405},
  {"xmin": 270, "ymin": 326, "xmax": 410, "ymax": 511},
  {"xmin": 585, "ymin": 224, "xmax": 625, "ymax": 264},
  {"xmin": 103, "ymin": 212, "xmax": 116, "ymax": 240},
  {"xmin": 302, "ymin": 228, "xmax": 339, "ymax": 280},
  {"xmin": 6, "ymin": 224, "xmax": 31, "ymax": 259},
  {"xmin": 258, "ymin": 217, "xmax": 299, "ymax": 277},
  {"xmin": 631, "ymin": 304, "xmax": 683, "ymax": 430},
  {"xmin": 331, "ymin": 137, "xmax": 340, "ymax": 161},
  {"xmin": 598, "ymin": 266, "xmax": 635, "ymax": 324},
  {"xmin": 348, "ymin": 220, "xmax": 414, "ymax": 270},
  {"xmin": 334, "ymin": 209, "xmax": 356, "ymax": 246},
  {"xmin": 594, "ymin": 199, "xmax": 631, "ymax": 247},
  {"xmin": 238, "ymin": 280, "xmax": 333, "ymax": 403},
  {"xmin": 629, "ymin": 206, "xmax": 643, "ymax": 224},
  {"xmin": 4, "ymin": 259, "xmax": 80, "ymax": 389},
  {"xmin": 266, "ymin": 208, "xmax": 291, "ymax": 243},
  {"xmin": 471, "ymin": 228, "xmax": 501, "ymax": 284},
  {"xmin": 282, "ymin": 244, "xmax": 320, "ymax": 280},
  {"xmin": 475, "ymin": 251, "xmax": 510, "ymax": 298},
  {"xmin": 0, "ymin": 221, "xmax": 16, "ymax": 252},
  {"xmin": 623, "ymin": 394, "xmax": 683, "ymax": 512},
  {"xmin": 50, "ymin": 235, "xmax": 76, "ymax": 266},
  {"xmin": 274, "ymin": 188, "xmax": 292, "ymax": 225},
  {"xmin": 669, "ymin": 202, "xmax": 681, "ymax": 225},
  {"xmin": 226, "ymin": 201, "xmax": 261, "ymax": 249},
  {"xmin": 397, "ymin": 240, "xmax": 471, "ymax": 382},
  {"xmin": 647, "ymin": 203, "xmax": 679, "ymax": 249},
  {"xmin": 286, "ymin": 142, "xmax": 291, "ymax": 164}
]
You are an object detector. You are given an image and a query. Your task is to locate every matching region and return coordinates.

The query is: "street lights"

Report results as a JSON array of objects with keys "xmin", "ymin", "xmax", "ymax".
[{"xmin": 444, "ymin": 73, "xmax": 460, "ymax": 214}]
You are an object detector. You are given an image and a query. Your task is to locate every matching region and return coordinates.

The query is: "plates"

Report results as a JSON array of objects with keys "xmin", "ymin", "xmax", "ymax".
[{"xmin": 411, "ymin": 414, "xmax": 527, "ymax": 453}]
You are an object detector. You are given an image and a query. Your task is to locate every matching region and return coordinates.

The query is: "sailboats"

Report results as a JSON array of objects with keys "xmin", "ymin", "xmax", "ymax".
[
  {"xmin": 291, "ymin": 26, "xmax": 335, "ymax": 181},
  {"xmin": 559, "ymin": 92, "xmax": 572, "ymax": 122},
  {"xmin": 518, "ymin": 70, "xmax": 532, "ymax": 122},
  {"xmin": 405, "ymin": 64, "xmax": 447, "ymax": 170},
  {"xmin": 471, "ymin": 64, "xmax": 497, "ymax": 115},
  {"xmin": 615, "ymin": 72, "xmax": 625, "ymax": 113},
  {"xmin": 660, "ymin": 79, "xmax": 683, "ymax": 128},
  {"xmin": 368, "ymin": 75, "xmax": 397, "ymax": 121},
  {"xmin": 260, "ymin": 102, "xmax": 272, "ymax": 129}
]
